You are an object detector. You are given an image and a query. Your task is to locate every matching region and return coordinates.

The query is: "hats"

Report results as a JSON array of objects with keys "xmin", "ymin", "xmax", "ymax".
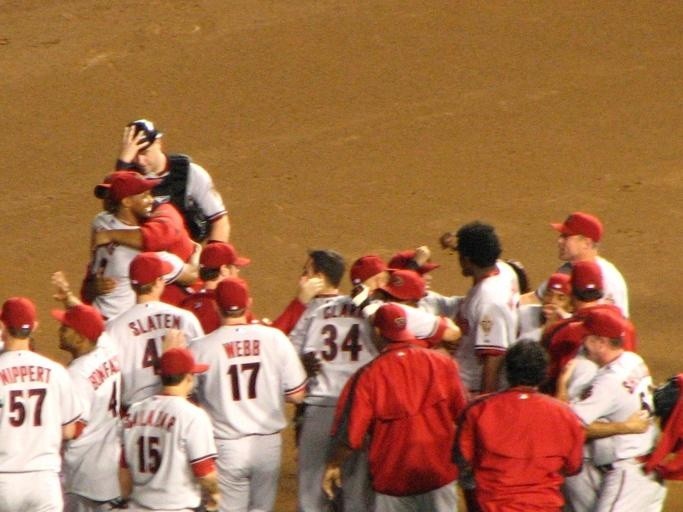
[
  {"xmin": 545, "ymin": 213, "xmax": 629, "ymax": 339},
  {"xmin": 48, "ymin": 305, "xmax": 106, "ymax": 344},
  {"xmin": 1, "ymin": 297, "xmax": 36, "ymax": 329},
  {"xmin": 351, "ymin": 251, "xmax": 440, "ymax": 342},
  {"xmin": 93, "ymin": 121, "xmax": 250, "ymax": 311},
  {"xmin": 159, "ymin": 349, "xmax": 208, "ymax": 375}
]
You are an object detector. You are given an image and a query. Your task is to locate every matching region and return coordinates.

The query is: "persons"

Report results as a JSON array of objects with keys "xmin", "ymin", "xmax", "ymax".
[
  {"xmin": 113, "ymin": 119, "xmax": 231, "ymax": 243},
  {"xmin": 79, "ymin": 170, "xmax": 203, "ymax": 321},
  {"xmin": 1, "ymin": 211, "xmax": 682, "ymax": 511}
]
